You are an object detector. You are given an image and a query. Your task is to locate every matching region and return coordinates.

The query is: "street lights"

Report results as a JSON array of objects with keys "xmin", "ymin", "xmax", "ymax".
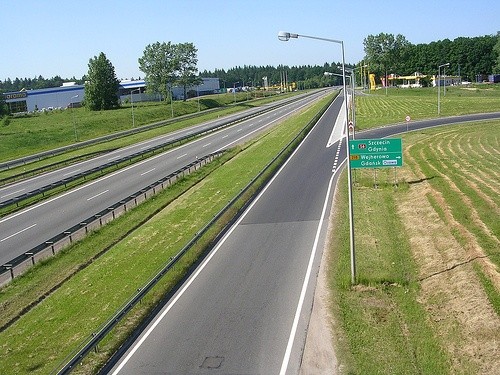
[
  {"xmin": 277, "ymin": 32, "xmax": 356, "ymax": 285},
  {"xmin": 437, "ymin": 62, "xmax": 462, "ymax": 115},
  {"xmin": 131, "ymin": 87, "xmax": 141, "ymax": 128},
  {"xmin": 168, "ymin": 75, "xmax": 272, "ymax": 117},
  {"xmin": 71, "ymin": 94, "xmax": 79, "ymax": 143}
]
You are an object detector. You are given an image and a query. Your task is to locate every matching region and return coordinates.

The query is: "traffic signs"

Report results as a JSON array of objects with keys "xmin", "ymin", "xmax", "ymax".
[{"xmin": 349, "ymin": 138, "xmax": 403, "ymax": 168}]
